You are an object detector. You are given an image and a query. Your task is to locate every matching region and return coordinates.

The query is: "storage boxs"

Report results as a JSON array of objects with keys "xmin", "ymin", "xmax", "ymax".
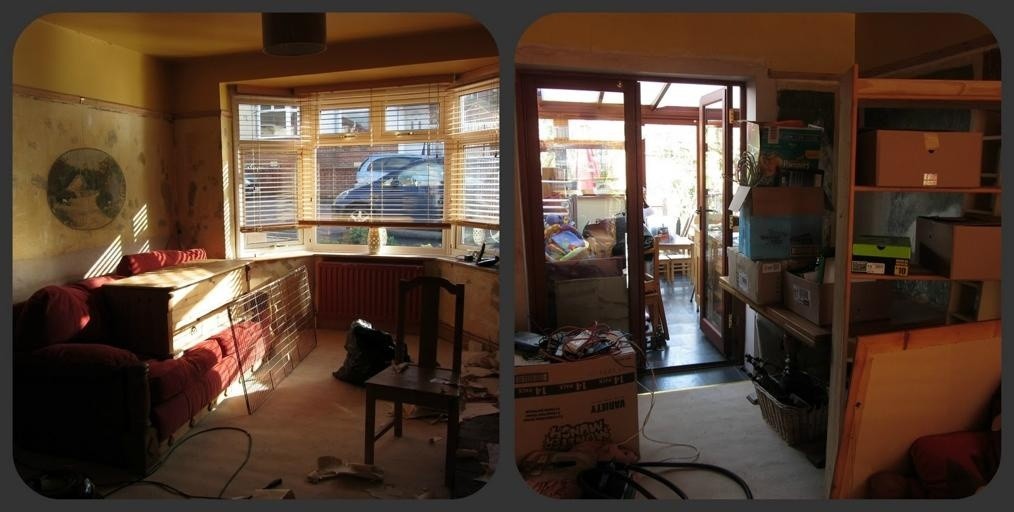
[
  {"xmin": 514, "ymin": 331, "xmax": 638, "ymax": 464},
  {"xmin": 728, "ymin": 186, "xmax": 835, "ymax": 259},
  {"xmin": 858, "ymin": 130, "xmax": 982, "ymax": 189},
  {"xmin": 786, "ymin": 271, "xmax": 893, "ymax": 325},
  {"xmin": 746, "ymin": 121, "xmax": 824, "ymax": 185},
  {"xmin": 727, "ymin": 247, "xmax": 815, "ymax": 305},
  {"xmin": 917, "ymin": 216, "xmax": 1001, "ymax": 280},
  {"xmin": 852, "ymin": 235, "xmax": 911, "ymax": 278}
]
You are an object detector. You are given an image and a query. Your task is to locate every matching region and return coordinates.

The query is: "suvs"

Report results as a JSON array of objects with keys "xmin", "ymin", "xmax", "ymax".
[{"xmin": 354, "ymin": 152, "xmax": 484, "ymax": 189}]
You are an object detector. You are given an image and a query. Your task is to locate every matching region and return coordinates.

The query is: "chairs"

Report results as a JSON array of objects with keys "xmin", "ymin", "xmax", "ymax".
[
  {"xmin": 644, "ymin": 236, "xmax": 670, "ymax": 340},
  {"xmin": 362, "ymin": 274, "xmax": 466, "ymax": 490},
  {"xmin": 644, "ymin": 293, "xmax": 658, "ymax": 338},
  {"xmin": 670, "ymin": 235, "xmax": 694, "ymax": 283},
  {"xmin": 649, "ymin": 255, "xmax": 670, "ymax": 283}
]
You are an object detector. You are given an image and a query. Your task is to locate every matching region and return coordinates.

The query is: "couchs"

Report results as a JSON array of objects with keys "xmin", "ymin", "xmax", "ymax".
[{"xmin": 13, "ymin": 249, "xmax": 273, "ymax": 479}]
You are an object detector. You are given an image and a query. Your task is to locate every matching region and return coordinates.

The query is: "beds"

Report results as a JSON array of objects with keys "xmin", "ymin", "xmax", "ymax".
[{"xmin": 658, "ymin": 235, "xmax": 694, "ymax": 278}]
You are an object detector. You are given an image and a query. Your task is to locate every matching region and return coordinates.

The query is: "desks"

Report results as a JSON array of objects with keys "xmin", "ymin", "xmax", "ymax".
[{"xmin": 718, "ymin": 276, "xmax": 946, "ymax": 445}]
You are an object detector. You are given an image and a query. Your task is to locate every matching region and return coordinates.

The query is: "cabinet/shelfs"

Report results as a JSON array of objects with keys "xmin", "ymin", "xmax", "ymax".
[
  {"xmin": 855, "ymin": 76, "xmax": 1002, "ymax": 361},
  {"xmin": 542, "ymin": 180, "xmax": 576, "ymax": 214},
  {"xmin": 102, "ymin": 259, "xmax": 254, "ymax": 359},
  {"xmin": 695, "ymin": 230, "xmax": 727, "ymax": 304},
  {"xmin": 946, "ymin": 48, "xmax": 1002, "ymax": 324}
]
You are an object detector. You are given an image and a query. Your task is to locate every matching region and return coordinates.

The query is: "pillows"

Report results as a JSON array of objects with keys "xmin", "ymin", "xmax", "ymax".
[{"xmin": 911, "ymin": 432, "xmax": 999, "ymax": 498}]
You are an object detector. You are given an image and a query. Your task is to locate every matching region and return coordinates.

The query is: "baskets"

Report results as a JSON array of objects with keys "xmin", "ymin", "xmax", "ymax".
[{"xmin": 751, "ymin": 368, "xmax": 831, "ymax": 451}]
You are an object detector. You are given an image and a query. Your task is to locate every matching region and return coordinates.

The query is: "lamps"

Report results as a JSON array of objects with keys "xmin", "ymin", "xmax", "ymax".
[{"xmin": 262, "ymin": 12, "xmax": 327, "ymax": 58}]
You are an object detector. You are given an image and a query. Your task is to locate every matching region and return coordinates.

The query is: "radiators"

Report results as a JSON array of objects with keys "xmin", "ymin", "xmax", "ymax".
[{"xmin": 315, "ymin": 262, "xmax": 422, "ymax": 335}]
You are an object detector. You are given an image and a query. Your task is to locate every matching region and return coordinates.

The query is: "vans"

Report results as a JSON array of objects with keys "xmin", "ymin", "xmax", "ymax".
[{"xmin": 333, "ymin": 156, "xmax": 499, "ymax": 247}]
